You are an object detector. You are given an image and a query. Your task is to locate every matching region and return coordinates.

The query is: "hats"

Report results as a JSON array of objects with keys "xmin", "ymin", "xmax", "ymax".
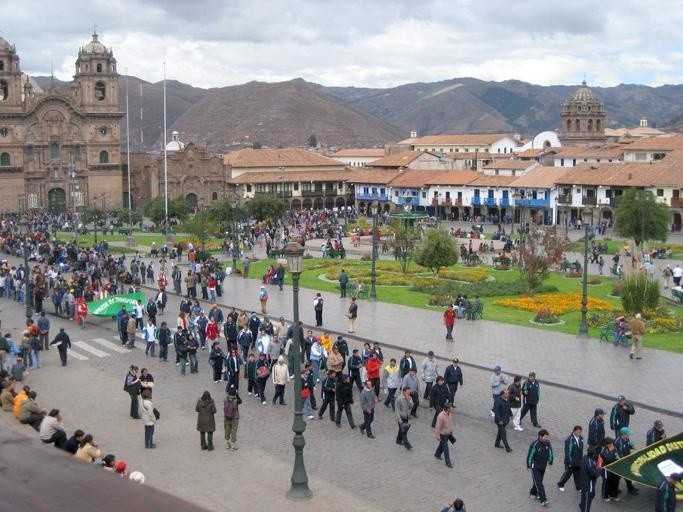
[
  {"xmin": 654, "ymin": 420, "xmax": 663, "ymax": 427},
  {"xmin": 493, "ymin": 365, "xmax": 501, "ymax": 372},
  {"xmin": 341, "ymin": 375, "xmax": 349, "ymax": 380},
  {"xmin": 452, "ymin": 358, "xmax": 458, "ymax": 363},
  {"xmin": 130, "ymin": 471, "xmax": 144, "ymax": 484},
  {"xmin": 118, "ymin": 462, "xmax": 125, "ymax": 470},
  {"xmin": 443, "ymin": 402, "xmax": 452, "ymax": 410},
  {"xmin": 616, "ymin": 395, "xmax": 625, "ymax": 402},
  {"xmin": 594, "ymin": 408, "xmax": 606, "ymax": 416},
  {"xmin": 620, "ymin": 427, "xmax": 633, "ymax": 436},
  {"xmin": 259, "ymin": 352, "xmax": 264, "ymax": 356}
]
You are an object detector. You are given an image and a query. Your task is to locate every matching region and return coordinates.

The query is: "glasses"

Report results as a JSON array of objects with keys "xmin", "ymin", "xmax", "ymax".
[
  {"xmin": 304, "ymin": 373, "xmax": 308, "ymax": 375},
  {"xmin": 134, "ymin": 370, "xmax": 137, "ymax": 373},
  {"xmin": 231, "ymin": 351, "xmax": 235, "ymax": 353}
]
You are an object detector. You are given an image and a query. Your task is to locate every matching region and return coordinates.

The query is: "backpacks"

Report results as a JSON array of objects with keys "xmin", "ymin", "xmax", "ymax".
[
  {"xmin": 31, "ymin": 324, "xmax": 39, "ymax": 336},
  {"xmin": 226, "ymin": 398, "xmax": 237, "ymax": 421}
]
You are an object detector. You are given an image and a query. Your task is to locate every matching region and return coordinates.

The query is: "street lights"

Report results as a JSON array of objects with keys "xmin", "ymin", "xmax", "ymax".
[
  {"xmin": 369, "ymin": 203, "xmax": 378, "ymax": 296},
  {"xmin": 230, "ymin": 201, "xmax": 237, "ymax": 273},
  {"xmin": 578, "ymin": 205, "xmax": 591, "ymax": 337},
  {"xmin": 18, "ymin": 218, "xmax": 35, "ymax": 323},
  {"xmin": 92, "ymin": 196, "xmax": 97, "ymax": 245},
  {"xmin": 282, "ymin": 242, "xmax": 310, "ymax": 497}
]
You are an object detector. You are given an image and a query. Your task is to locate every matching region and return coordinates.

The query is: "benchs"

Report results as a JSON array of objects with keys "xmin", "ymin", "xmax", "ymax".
[
  {"xmin": 670, "ymin": 284, "xmax": 682, "ymax": 305},
  {"xmin": 451, "ymin": 301, "xmax": 483, "ymax": 321},
  {"xmin": 263, "ymin": 270, "xmax": 283, "ymax": 285},
  {"xmin": 267, "ymin": 246, "xmax": 283, "ymax": 256},
  {"xmin": 342, "ymin": 281, "xmax": 368, "ymax": 300},
  {"xmin": 322, "ymin": 247, "xmax": 345, "ymax": 258},
  {"xmin": 490, "ymin": 254, "xmax": 513, "ymax": 268},
  {"xmin": 560, "ymin": 259, "xmax": 581, "ymax": 273},
  {"xmin": 459, "ymin": 249, "xmax": 482, "ymax": 267},
  {"xmin": 597, "ymin": 321, "xmax": 637, "ymax": 349},
  {"xmin": 609, "ymin": 265, "xmax": 624, "ymax": 280}
]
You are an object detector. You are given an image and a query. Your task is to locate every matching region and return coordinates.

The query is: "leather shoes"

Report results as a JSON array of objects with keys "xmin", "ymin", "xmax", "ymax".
[
  {"xmin": 201, "ymin": 445, "xmax": 207, "ymax": 449},
  {"xmin": 312, "ymin": 406, "xmax": 318, "ymax": 410},
  {"xmin": 367, "ymin": 435, "xmax": 376, "ymax": 438},
  {"xmin": 351, "ymin": 425, "xmax": 357, "ymax": 429},
  {"xmin": 534, "ymin": 424, "xmax": 541, "ymax": 428},
  {"xmin": 505, "ymin": 448, "xmax": 512, "ymax": 452},
  {"xmin": 209, "ymin": 445, "xmax": 213, "ymax": 450},
  {"xmin": 318, "ymin": 414, "xmax": 322, "ymax": 419},
  {"xmin": 335, "ymin": 422, "xmax": 342, "ymax": 428},
  {"xmin": 358, "ymin": 424, "xmax": 365, "ymax": 434},
  {"xmin": 433, "ymin": 454, "xmax": 443, "ymax": 460},
  {"xmin": 628, "ymin": 484, "xmax": 640, "ymax": 494},
  {"xmin": 330, "ymin": 418, "xmax": 335, "ymax": 421},
  {"xmin": 445, "ymin": 463, "xmax": 452, "ymax": 467},
  {"xmin": 494, "ymin": 445, "xmax": 504, "ymax": 448}
]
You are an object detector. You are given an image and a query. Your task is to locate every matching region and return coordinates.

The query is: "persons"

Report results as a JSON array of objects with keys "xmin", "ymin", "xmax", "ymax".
[
  {"xmin": 189, "ymin": 248, "xmax": 195, "ymax": 266},
  {"xmin": 260, "ymin": 286, "xmax": 267, "ymax": 313},
  {"xmin": 219, "ymin": 267, "xmax": 224, "ymax": 295},
  {"xmin": 208, "ymin": 342, "xmax": 227, "ymax": 381},
  {"xmin": 0, "ymin": 208, "xmax": 72, "ymax": 265},
  {"xmin": 136, "ymin": 299, "xmax": 144, "ymax": 331},
  {"xmin": 210, "ymin": 305, "xmax": 221, "ymax": 325},
  {"xmin": 177, "ymin": 245, "xmax": 184, "ymax": 261},
  {"xmin": 556, "ymin": 426, "xmax": 584, "ymax": 490},
  {"xmin": 657, "ymin": 472, "xmax": 679, "ymax": 511},
  {"xmin": 326, "ymin": 346, "xmax": 345, "ymax": 374},
  {"xmin": 171, "ymin": 265, "xmax": 175, "ymax": 290},
  {"xmin": 38, "ymin": 312, "xmax": 50, "ymax": 350},
  {"xmin": 495, "ymin": 390, "xmax": 514, "ymax": 453},
  {"xmin": 173, "ymin": 326, "xmax": 190, "ymax": 367},
  {"xmin": 176, "ymin": 268, "xmax": 182, "ymax": 296},
  {"xmin": 521, "ymin": 372, "xmax": 542, "ymax": 428},
  {"xmin": 303, "ymin": 329, "xmax": 314, "ymax": 359},
  {"xmin": 21, "ymin": 330, "xmax": 33, "ymax": 375},
  {"xmin": 441, "ymin": 208, "xmax": 530, "ymax": 269},
  {"xmin": 276, "ymin": 263, "xmax": 286, "ymax": 290},
  {"xmin": 127, "ymin": 471, "xmax": 147, "ymax": 485},
  {"xmin": 121, "ymin": 312, "xmax": 129, "ymax": 344},
  {"xmin": 598, "ymin": 438, "xmax": 623, "ymax": 502},
  {"xmin": 52, "ymin": 327, "xmax": 72, "ymax": 368},
  {"xmin": 70, "ymin": 219, "xmax": 181, "ymax": 236},
  {"xmin": 366, "ymin": 352, "xmax": 385, "ymax": 403},
  {"xmin": 401, "ymin": 349, "xmax": 414, "ymax": 374},
  {"xmin": 334, "ymin": 334, "xmax": 351, "ymax": 362},
  {"xmin": 299, "ymin": 321, "xmax": 307, "ymax": 364},
  {"xmin": 286, "ymin": 207, "xmax": 365, "ymax": 259},
  {"xmin": 420, "ymin": 217, "xmax": 437, "ymax": 230},
  {"xmin": 508, "ymin": 377, "xmax": 524, "ymax": 431},
  {"xmin": 614, "ymin": 315, "xmax": 626, "ymax": 347},
  {"xmin": 311, "ymin": 338, "xmax": 321, "ymax": 390},
  {"xmin": 170, "ymin": 249, "xmax": 178, "ymax": 267},
  {"xmin": 243, "ymin": 353, "xmax": 259, "ymax": 398},
  {"xmin": 302, "ymin": 371, "xmax": 314, "ymax": 420},
  {"xmin": 181, "ymin": 329, "xmax": 189, "ymax": 337},
  {"xmin": 443, "ymin": 359, "xmax": 464, "ymax": 401},
  {"xmin": 526, "ymin": 430, "xmax": 555, "ymax": 506},
  {"xmin": 156, "ymin": 322, "xmax": 172, "ymax": 365},
  {"xmin": 126, "ymin": 315, "xmax": 138, "ymax": 349},
  {"xmin": 6, "ymin": 333, "xmax": 21, "ymax": 356},
  {"xmin": 356, "ymin": 280, "xmax": 366, "ymax": 299},
  {"xmin": 630, "ymin": 312, "xmax": 646, "ymax": 362},
  {"xmin": 226, "ymin": 318, "xmax": 237, "ymax": 348},
  {"xmin": 13, "ymin": 386, "xmax": 30, "ymax": 417},
  {"xmin": 538, "ymin": 213, "xmax": 552, "ymax": 225},
  {"xmin": 200, "ymin": 274, "xmax": 208, "ymax": 298},
  {"xmin": 38, "ymin": 408, "xmax": 68, "ymax": 450},
  {"xmin": 467, "ymin": 295, "xmax": 480, "ymax": 320},
  {"xmin": 12, "ymin": 359, "xmax": 29, "ymax": 387},
  {"xmin": 322, "ymin": 329, "xmax": 333, "ymax": 365},
  {"xmin": 144, "ymin": 318, "xmax": 158, "ymax": 359},
  {"xmin": 376, "ymin": 207, "xmax": 395, "ymax": 229},
  {"xmin": 221, "ymin": 218, "xmax": 270, "ymax": 253},
  {"xmin": 214, "ymin": 269, "xmax": 222, "ymax": 296},
  {"xmin": 457, "ymin": 295, "xmax": 470, "ymax": 318},
  {"xmin": 358, "ymin": 379, "xmax": 377, "ymax": 440},
  {"xmin": 186, "ymin": 334, "xmax": 200, "ymax": 377},
  {"xmin": 242, "ymin": 256, "xmax": 250, "ymax": 276},
  {"xmin": 102, "ymin": 455, "xmax": 114, "ymax": 470},
  {"xmin": 313, "ymin": 293, "xmax": 323, "ymax": 327},
  {"xmin": 337, "ymin": 370, "xmax": 356, "ymax": 430},
  {"xmin": 278, "ymin": 315, "xmax": 289, "ymax": 341},
  {"xmin": 257, "ymin": 328, "xmax": 273, "ymax": 357},
  {"xmin": 210, "ymin": 315, "xmax": 220, "ymax": 352},
  {"xmin": 156, "ymin": 269, "xmax": 167, "ymax": 289},
  {"xmin": 115, "ymin": 462, "xmax": 127, "ymax": 474},
  {"xmin": 0, "ymin": 333, "xmax": 9, "ymax": 367},
  {"xmin": 193, "ymin": 258, "xmax": 219, "ymax": 281},
  {"xmin": 319, "ymin": 372, "xmax": 337, "ymax": 425},
  {"xmin": 249, "ymin": 314, "xmax": 259, "ymax": 328},
  {"xmin": 434, "ymin": 402, "xmax": 458, "ymax": 470},
  {"xmin": 429, "ymin": 376, "xmax": 452, "ymax": 427},
  {"xmin": 178, "ymin": 337, "xmax": 190, "ymax": 377},
  {"xmin": 422, "ymin": 352, "xmax": 438, "ymax": 401},
  {"xmin": 382, "ymin": 360, "xmax": 401, "ymax": 409},
  {"xmin": 616, "ymin": 427, "xmax": 641, "ymax": 496},
  {"xmin": 117, "ymin": 304, "xmax": 129, "ymax": 341},
  {"xmin": 236, "ymin": 324, "xmax": 253, "ymax": 361},
  {"xmin": 348, "ymin": 297, "xmax": 357, "ymax": 332},
  {"xmin": 160, "ymin": 268, "xmax": 168, "ymax": 291},
  {"xmin": 262, "ymin": 316, "xmax": 275, "ymax": 334},
  {"xmin": 71, "ymin": 240, "xmax": 158, "ymax": 294},
  {"xmin": 443, "ymin": 304, "xmax": 457, "ymax": 341},
  {"xmin": 266, "ymin": 216, "xmax": 289, "ymax": 251},
  {"xmin": 177, "ymin": 311, "xmax": 188, "ymax": 334},
  {"xmin": 230, "ymin": 304, "xmax": 238, "ymax": 315},
  {"xmin": 160, "ymin": 244, "xmax": 169, "ymax": 261},
  {"xmin": 31, "ymin": 332, "xmax": 42, "ymax": 370},
  {"xmin": 0, "ymin": 262, "xmax": 80, "ymax": 320},
  {"xmin": 362, "ymin": 342, "xmax": 371, "ymax": 358},
  {"xmin": 188, "ymin": 272, "xmax": 198, "ymax": 301},
  {"xmin": 137, "ymin": 387, "xmax": 162, "ymax": 449},
  {"xmin": 123, "ymin": 366, "xmax": 142, "ymax": 419},
  {"xmin": 181, "ymin": 294, "xmax": 192, "ymax": 315},
  {"xmin": 197, "ymin": 391, "xmax": 216, "ymax": 453},
  {"xmin": 139, "ymin": 369, "xmax": 154, "ymax": 399},
  {"xmin": 454, "ymin": 296, "xmax": 461, "ymax": 310},
  {"xmin": 395, "ymin": 386, "xmax": 414, "ymax": 452},
  {"xmin": 229, "ymin": 349, "xmax": 242, "ymax": 386},
  {"xmin": 272, "ymin": 354, "xmax": 290, "ymax": 406},
  {"xmin": 76, "ymin": 436, "xmax": 101, "ymax": 462},
  {"xmin": 195, "ymin": 317, "xmax": 208, "ymax": 351},
  {"xmin": 611, "ymin": 396, "xmax": 631, "ymax": 437},
  {"xmin": 646, "ymin": 422, "xmax": 667, "ymax": 445},
  {"xmin": 444, "ymin": 500, "xmax": 468, "ymax": 510},
  {"xmin": 238, "ymin": 310, "xmax": 248, "ymax": 322},
  {"xmin": 262, "ymin": 266, "xmax": 275, "ymax": 284},
  {"xmin": 373, "ymin": 343, "xmax": 381, "ymax": 357},
  {"xmin": 148, "ymin": 296, "xmax": 158, "ymax": 326},
  {"xmin": 208, "ymin": 276, "xmax": 217, "ymax": 301},
  {"xmin": 306, "ymin": 364, "xmax": 319, "ymax": 410},
  {"xmin": 2, "ymin": 370, "xmax": 11, "ymax": 387},
  {"xmin": 490, "ymin": 365, "xmax": 509, "ymax": 415},
  {"xmin": 268, "ymin": 335, "xmax": 282, "ymax": 359},
  {"xmin": 189, "ymin": 269, "xmax": 198, "ymax": 288},
  {"xmin": 401, "ymin": 370, "xmax": 418, "ymax": 420},
  {"xmin": 347, "ymin": 349, "xmax": 363, "ymax": 371},
  {"xmin": 1, "ymin": 381, "xmax": 16, "ymax": 410},
  {"xmin": 154, "ymin": 287, "xmax": 166, "ymax": 313},
  {"xmin": 66, "ymin": 430, "xmax": 85, "ymax": 454},
  {"xmin": 563, "ymin": 216, "xmax": 683, "ymax": 289},
  {"xmin": 338, "ymin": 268, "xmax": 347, "ymax": 297},
  {"xmin": 19, "ymin": 393, "xmax": 44, "ymax": 433},
  {"xmin": 575, "ymin": 448, "xmax": 598, "ymax": 511},
  {"xmin": 224, "ymin": 387, "xmax": 242, "ymax": 451},
  {"xmin": 589, "ymin": 408, "xmax": 606, "ymax": 444},
  {"xmin": 193, "ymin": 300, "xmax": 202, "ymax": 313},
  {"xmin": 27, "ymin": 320, "xmax": 41, "ymax": 337}
]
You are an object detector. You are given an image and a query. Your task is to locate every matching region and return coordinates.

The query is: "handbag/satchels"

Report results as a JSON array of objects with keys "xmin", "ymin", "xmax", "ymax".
[
  {"xmin": 448, "ymin": 434, "xmax": 456, "ymax": 444},
  {"xmin": 346, "ymin": 303, "xmax": 355, "ymax": 318},
  {"xmin": 300, "ymin": 379, "xmax": 311, "ymax": 399},
  {"xmin": 207, "ymin": 350, "xmax": 216, "ymax": 368},
  {"xmin": 141, "ymin": 400, "xmax": 159, "ymax": 421},
  {"xmin": 399, "ymin": 412, "xmax": 411, "ymax": 433},
  {"xmin": 164, "ymin": 330, "xmax": 172, "ymax": 344}
]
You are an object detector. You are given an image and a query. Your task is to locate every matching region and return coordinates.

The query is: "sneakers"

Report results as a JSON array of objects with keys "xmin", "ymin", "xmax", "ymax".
[
  {"xmin": 279, "ymin": 402, "xmax": 287, "ymax": 405},
  {"xmin": 226, "ymin": 443, "xmax": 230, "ymax": 448},
  {"xmin": 610, "ymin": 496, "xmax": 619, "ymax": 501},
  {"xmin": 602, "ymin": 498, "xmax": 610, "ymax": 502},
  {"xmin": 271, "ymin": 400, "xmax": 276, "ymax": 405},
  {"xmin": 263, "ymin": 401, "xmax": 266, "ymax": 405},
  {"xmin": 576, "ymin": 503, "xmax": 584, "ymax": 511},
  {"xmin": 529, "ymin": 494, "xmax": 540, "ymax": 500},
  {"xmin": 212, "ymin": 381, "xmax": 218, "ymax": 384},
  {"xmin": 216, "ymin": 379, "xmax": 222, "ymax": 382},
  {"xmin": 395, "ymin": 441, "xmax": 405, "ymax": 445},
  {"xmin": 540, "ymin": 500, "xmax": 550, "ymax": 507},
  {"xmin": 175, "ymin": 361, "xmax": 179, "ymax": 366},
  {"xmin": 559, "ymin": 486, "xmax": 564, "ymax": 491},
  {"xmin": 405, "ymin": 444, "xmax": 413, "ymax": 449},
  {"xmin": 248, "ymin": 392, "xmax": 252, "ymax": 395},
  {"xmin": 513, "ymin": 427, "xmax": 524, "ymax": 432},
  {"xmin": 255, "ymin": 393, "xmax": 258, "ymax": 397},
  {"xmin": 579, "ymin": 489, "xmax": 582, "ymax": 492},
  {"xmin": 184, "ymin": 362, "xmax": 189, "ymax": 366},
  {"xmin": 490, "ymin": 409, "xmax": 495, "ymax": 417},
  {"xmin": 307, "ymin": 415, "xmax": 314, "ymax": 420},
  {"xmin": 231, "ymin": 445, "xmax": 238, "ymax": 450}
]
